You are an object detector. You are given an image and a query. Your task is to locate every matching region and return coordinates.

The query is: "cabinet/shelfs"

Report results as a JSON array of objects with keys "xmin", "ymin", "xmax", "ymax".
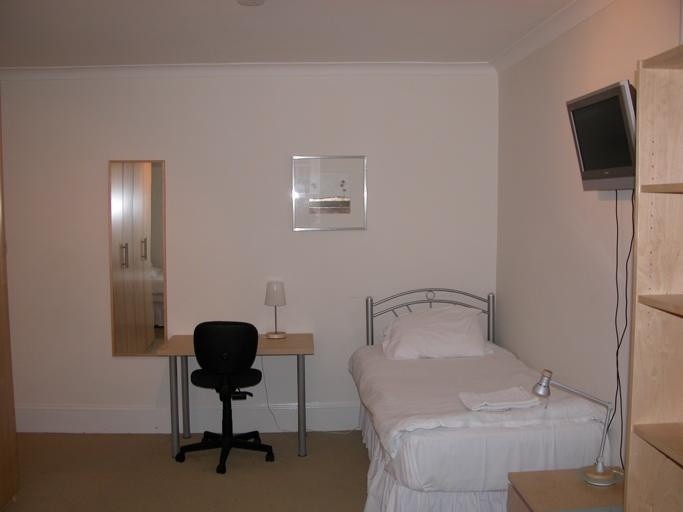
[
  {"xmin": 506, "ymin": 44, "xmax": 683, "ymax": 511},
  {"xmin": 112, "ymin": 163, "xmax": 155, "ymax": 354}
]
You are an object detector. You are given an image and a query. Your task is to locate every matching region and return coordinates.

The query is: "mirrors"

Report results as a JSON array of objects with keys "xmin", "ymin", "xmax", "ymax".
[{"xmin": 107, "ymin": 160, "xmax": 167, "ymax": 357}]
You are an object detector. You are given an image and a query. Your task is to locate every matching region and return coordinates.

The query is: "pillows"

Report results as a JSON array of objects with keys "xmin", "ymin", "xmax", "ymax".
[{"xmin": 382, "ymin": 307, "xmax": 494, "ymax": 360}]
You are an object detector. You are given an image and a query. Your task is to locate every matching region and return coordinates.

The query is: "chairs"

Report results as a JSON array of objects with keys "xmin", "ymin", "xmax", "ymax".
[{"xmin": 175, "ymin": 321, "xmax": 274, "ymax": 474}]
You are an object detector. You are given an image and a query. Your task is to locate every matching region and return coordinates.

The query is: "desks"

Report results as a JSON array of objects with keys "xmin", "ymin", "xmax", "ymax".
[{"xmin": 155, "ymin": 333, "xmax": 314, "ymax": 458}]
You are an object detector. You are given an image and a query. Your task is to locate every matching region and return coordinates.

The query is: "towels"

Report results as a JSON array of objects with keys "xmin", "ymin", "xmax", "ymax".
[{"xmin": 460, "ymin": 386, "xmax": 540, "ymax": 411}]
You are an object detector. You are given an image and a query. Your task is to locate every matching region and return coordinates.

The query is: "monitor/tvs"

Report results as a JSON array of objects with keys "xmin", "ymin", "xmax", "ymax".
[{"xmin": 567, "ymin": 79, "xmax": 637, "ymax": 191}]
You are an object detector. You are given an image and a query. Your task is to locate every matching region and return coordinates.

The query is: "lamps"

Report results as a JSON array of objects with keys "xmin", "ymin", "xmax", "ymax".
[
  {"xmin": 265, "ymin": 281, "xmax": 286, "ymax": 339},
  {"xmin": 533, "ymin": 369, "xmax": 621, "ymax": 487}
]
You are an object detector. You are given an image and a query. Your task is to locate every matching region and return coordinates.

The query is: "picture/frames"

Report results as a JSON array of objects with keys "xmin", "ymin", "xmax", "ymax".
[{"xmin": 292, "ymin": 155, "xmax": 367, "ymax": 232}]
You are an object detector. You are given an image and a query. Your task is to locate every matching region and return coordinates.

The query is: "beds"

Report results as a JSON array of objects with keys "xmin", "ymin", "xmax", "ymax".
[{"xmin": 349, "ymin": 288, "xmax": 610, "ymax": 512}]
